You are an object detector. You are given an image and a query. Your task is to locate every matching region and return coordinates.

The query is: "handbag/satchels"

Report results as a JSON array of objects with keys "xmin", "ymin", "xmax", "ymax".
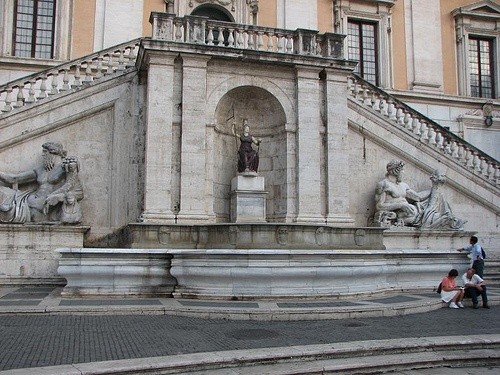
[
  {"xmin": 481, "ymin": 247, "xmax": 485, "ymax": 259},
  {"xmin": 437, "ymin": 282, "xmax": 442, "ymax": 294}
]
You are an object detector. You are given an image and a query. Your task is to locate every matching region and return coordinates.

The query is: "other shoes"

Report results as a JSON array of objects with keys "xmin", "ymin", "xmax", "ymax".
[
  {"xmin": 482, "ymin": 304, "xmax": 491, "ymax": 309},
  {"xmin": 473, "ymin": 303, "xmax": 479, "ymax": 309},
  {"xmin": 458, "ymin": 301, "xmax": 464, "ymax": 308},
  {"xmin": 449, "ymin": 303, "xmax": 459, "ymax": 308}
]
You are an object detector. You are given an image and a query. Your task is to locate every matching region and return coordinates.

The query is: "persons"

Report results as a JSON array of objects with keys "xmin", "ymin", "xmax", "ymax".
[
  {"xmin": 231, "ymin": 120, "xmax": 262, "ymax": 173},
  {"xmin": 62, "ymin": 192, "xmax": 84, "ymax": 224},
  {"xmin": 440, "ymin": 269, "xmax": 465, "ymax": 309},
  {"xmin": 456, "ymin": 236, "xmax": 484, "ymax": 279},
  {"xmin": 0, "ymin": 141, "xmax": 67, "ymax": 223},
  {"xmin": 374, "ymin": 159, "xmax": 432, "ymax": 225},
  {"xmin": 462, "ymin": 268, "xmax": 491, "ymax": 310}
]
